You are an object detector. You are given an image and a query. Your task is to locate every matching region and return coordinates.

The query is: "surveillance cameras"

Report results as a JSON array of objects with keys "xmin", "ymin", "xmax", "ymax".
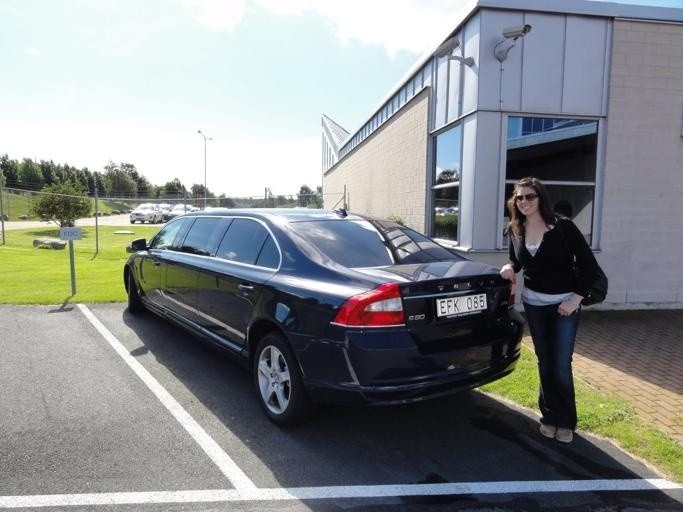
[
  {"xmin": 503, "ymin": 24, "xmax": 532, "ymax": 40},
  {"xmin": 432, "ymin": 38, "xmax": 460, "ymax": 58}
]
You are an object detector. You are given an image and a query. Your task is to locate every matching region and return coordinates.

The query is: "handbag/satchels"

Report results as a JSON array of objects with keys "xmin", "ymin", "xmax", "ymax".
[{"xmin": 583, "ymin": 267, "xmax": 608, "ymax": 305}]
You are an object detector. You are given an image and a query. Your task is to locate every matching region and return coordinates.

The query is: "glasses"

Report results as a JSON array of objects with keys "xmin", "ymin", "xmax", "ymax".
[{"xmin": 514, "ymin": 194, "xmax": 539, "ymax": 201}]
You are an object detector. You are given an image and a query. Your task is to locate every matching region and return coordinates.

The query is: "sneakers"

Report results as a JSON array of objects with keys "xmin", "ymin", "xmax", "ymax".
[
  {"xmin": 556, "ymin": 427, "xmax": 573, "ymax": 443},
  {"xmin": 540, "ymin": 424, "xmax": 555, "ymax": 439}
]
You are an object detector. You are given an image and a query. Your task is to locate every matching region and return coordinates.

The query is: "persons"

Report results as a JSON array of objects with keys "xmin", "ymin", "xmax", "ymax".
[{"xmin": 499, "ymin": 176, "xmax": 598, "ymax": 444}]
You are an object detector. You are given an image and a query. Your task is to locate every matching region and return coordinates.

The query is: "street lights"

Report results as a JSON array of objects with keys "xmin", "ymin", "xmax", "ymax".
[{"xmin": 198, "ymin": 130, "xmax": 213, "ymax": 211}]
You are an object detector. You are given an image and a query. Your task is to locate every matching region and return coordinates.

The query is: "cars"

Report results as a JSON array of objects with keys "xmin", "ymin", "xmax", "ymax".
[
  {"xmin": 129, "ymin": 202, "xmax": 201, "ymax": 225},
  {"xmin": 435, "ymin": 206, "xmax": 459, "ymax": 216},
  {"xmin": 122, "ymin": 184, "xmax": 527, "ymax": 431}
]
